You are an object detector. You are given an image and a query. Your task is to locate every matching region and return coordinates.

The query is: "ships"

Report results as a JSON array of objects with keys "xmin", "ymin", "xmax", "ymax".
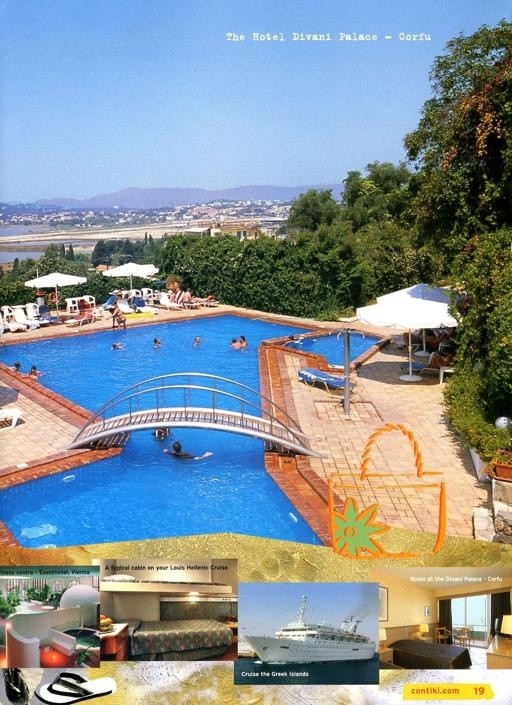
[{"xmin": 241, "ymin": 594, "xmax": 376, "ymax": 664}]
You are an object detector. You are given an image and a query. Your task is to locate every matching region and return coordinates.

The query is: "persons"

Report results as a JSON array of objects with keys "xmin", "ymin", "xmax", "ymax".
[
  {"xmin": 105, "ymin": 307, "xmax": 127, "ymax": 329},
  {"xmin": 11, "ymin": 361, "xmax": 41, "ymax": 382},
  {"xmin": 155, "ymin": 428, "xmax": 171, "ymax": 441},
  {"xmin": 112, "ymin": 342, "xmax": 121, "ymax": 349},
  {"xmin": 230, "ymin": 337, "xmax": 241, "ymax": 350},
  {"xmin": 47, "ymin": 289, "xmax": 56, "ymax": 311},
  {"xmin": 240, "ymin": 335, "xmax": 247, "ymax": 347},
  {"xmin": 281, "ymin": 335, "xmax": 296, "ymax": 349},
  {"xmin": 162, "ymin": 442, "xmax": 215, "ymax": 462},
  {"xmin": 153, "ymin": 338, "xmax": 160, "ymax": 345},
  {"xmin": 195, "ymin": 336, "xmax": 202, "ymax": 345},
  {"xmin": 403, "ymin": 326, "xmax": 456, "ymax": 369},
  {"xmin": 172, "ymin": 288, "xmax": 218, "ymax": 310}
]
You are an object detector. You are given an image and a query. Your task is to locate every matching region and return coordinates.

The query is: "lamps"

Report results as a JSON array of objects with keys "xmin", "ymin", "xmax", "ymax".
[
  {"xmin": 419, "ymin": 623, "xmax": 429, "ymax": 638},
  {"xmin": 379, "ymin": 628, "xmax": 387, "ymax": 650},
  {"xmin": 500, "ymin": 615, "xmax": 512, "ymax": 640}
]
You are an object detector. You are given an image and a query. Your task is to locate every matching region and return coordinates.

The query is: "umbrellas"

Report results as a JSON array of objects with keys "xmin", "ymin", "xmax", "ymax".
[
  {"xmin": 102, "ymin": 261, "xmax": 160, "ymax": 303},
  {"xmin": 356, "ymin": 295, "xmax": 463, "ymax": 376},
  {"xmin": 375, "ymin": 284, "xmax": 467, "ymax": 351},
  {"xmin": 25, "ymin": 272, "xmax": 87, "ymax": 318}
]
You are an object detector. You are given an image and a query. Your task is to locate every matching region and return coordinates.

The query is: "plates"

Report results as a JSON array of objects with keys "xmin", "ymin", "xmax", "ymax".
[{"xmin": 100, "ymin": 627, "xmax": 116, "ymax": 634}]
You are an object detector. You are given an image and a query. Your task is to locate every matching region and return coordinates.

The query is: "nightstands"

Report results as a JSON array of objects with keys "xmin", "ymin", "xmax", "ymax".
[
  {"xmin": 100, "ymin": 623, "xmax": 129, "ymax": 661},
  {"xmin": 379, "ymin": 647, "xmax": 394, "ymax": 666}
]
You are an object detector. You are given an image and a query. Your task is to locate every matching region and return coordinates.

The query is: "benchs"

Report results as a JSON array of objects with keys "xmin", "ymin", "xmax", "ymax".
[{"xmin": 298, "ymin": 368, "xmax": 357, "ymax": 395}]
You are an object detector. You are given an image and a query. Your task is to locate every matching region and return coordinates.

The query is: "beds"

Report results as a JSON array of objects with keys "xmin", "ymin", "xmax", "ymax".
[
  {"xmin": 112, "ymin": 618, "xmax": 233, "ymax": 661},
  {"xmin": 387, "ymin": 639, "xmax": 469, "ymax": 669}
]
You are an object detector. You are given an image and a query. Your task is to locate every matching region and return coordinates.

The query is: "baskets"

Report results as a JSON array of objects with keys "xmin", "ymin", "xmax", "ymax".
[{"xmin": 324, "ymin": 424, "xmax": 448, "ymax": 561}]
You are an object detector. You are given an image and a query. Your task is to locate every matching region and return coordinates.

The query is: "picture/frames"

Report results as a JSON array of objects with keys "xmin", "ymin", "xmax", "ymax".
[
  {"xmin": 378, "ymin": 586, "xmax": 388, "ymax": 623},
  {"xmin": 425, "ymin": 606, "xmax": 430, "ymax": 617}
]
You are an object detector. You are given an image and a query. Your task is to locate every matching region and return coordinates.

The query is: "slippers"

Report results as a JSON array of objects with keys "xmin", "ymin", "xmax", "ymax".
[{"xmin": 33, "ymin": 672, "xmax": 117, "ymax": 705}]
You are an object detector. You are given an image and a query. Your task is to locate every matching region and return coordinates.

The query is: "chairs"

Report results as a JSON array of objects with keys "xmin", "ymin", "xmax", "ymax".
[
  {"xmin": 433, "ymin": 627, "xmax": 471, "ymax": 651},
  {"xmin": 390, "ymin": 328, "xmax": 456, "ymax": 384},
  {"xmin": 1, "ymin": 407, "xmax": 23, "ymax": 428},
  {"xmin": 0, "ymin": 287, "xmax": 219, "ymax": 335}
]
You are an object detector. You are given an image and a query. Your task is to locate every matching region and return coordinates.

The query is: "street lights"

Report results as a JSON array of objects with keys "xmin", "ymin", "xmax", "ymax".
[{"xmin": 337, "ymin": 317, "xmax": 358, "ymax": 414}]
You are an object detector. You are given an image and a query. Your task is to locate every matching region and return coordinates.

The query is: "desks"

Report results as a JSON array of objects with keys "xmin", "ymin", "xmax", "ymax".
[{"xmin": 486, "ymin": 633, "xmax": 512, "ymax": 669}]
